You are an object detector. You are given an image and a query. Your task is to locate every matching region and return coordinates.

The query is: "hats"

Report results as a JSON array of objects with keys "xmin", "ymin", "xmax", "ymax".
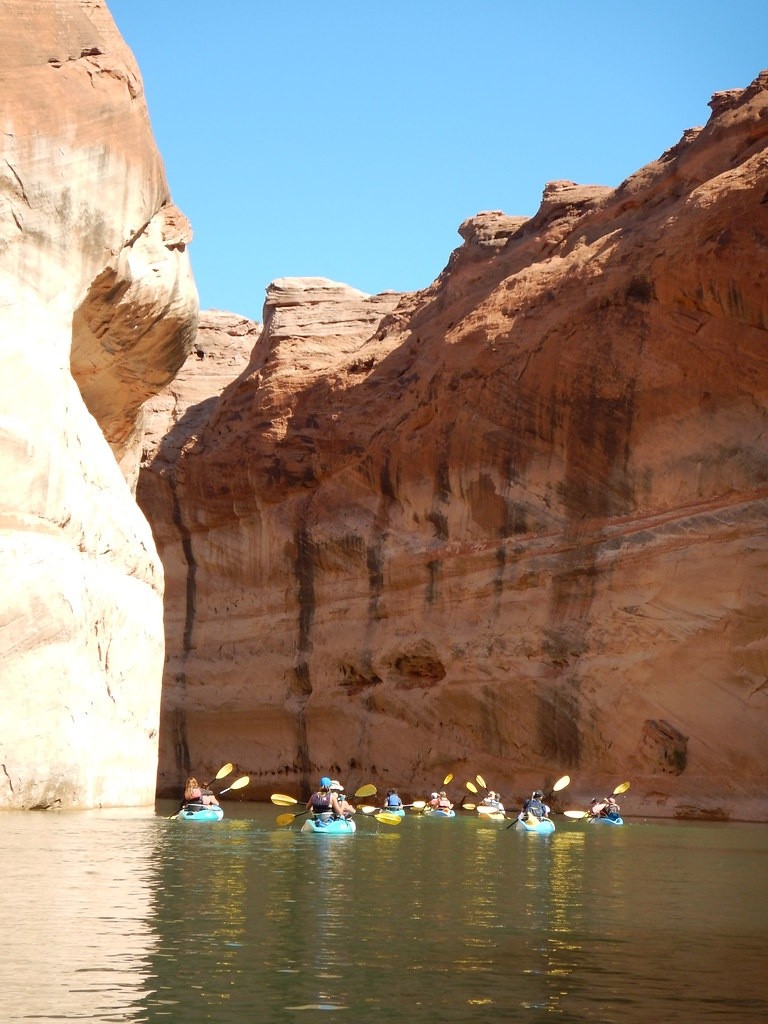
[
  {"xmin": 320, "ymin": 777, "xmax": 332, "ymax": 787},
  {"xmin": 329, "ymin": 780, "xmax": 344, "ymax": 792},
  {"xmin": 534, "ymin": 790, "xmax": 544, "ymax": 798}
]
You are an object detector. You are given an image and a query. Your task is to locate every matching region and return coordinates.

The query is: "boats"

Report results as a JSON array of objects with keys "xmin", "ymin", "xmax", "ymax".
[
  {"xmin": 378, "ymin": 809, "xmax": 405, "ymax": 816},
  {"xmin": 586, "ymin": 817, "xmax": 624, "ymax": 825},
  {"xmin": 176, "ymin": 804, "xmax": 224, "ymax": 821},
  {"xmin": 424, "ymin": 808, "xmax": 456, "ymax": 818},
  {"xmin": 514, "ymin": 815, "xmax": 555, "ymax": 832},
  {"xmin": 300, "ymin": 818, "xmax": 356, "ymax": 834},
  {"xmin": 477, "ymin": 811, "xmax": 504, "ymax": 819}
]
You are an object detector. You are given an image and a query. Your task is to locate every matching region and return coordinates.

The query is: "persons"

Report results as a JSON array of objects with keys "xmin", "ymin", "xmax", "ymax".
[
  {"xmin": 306, "ymin": 777, "xmax": 355, "ymax": 819},
  {"xmin": 384, "ymin": 788, "xmax": 505, "ymax": 814},
  {"xmin": 522, "ymin": 790, "xmax": 550, "ymax": 819},
  {"xmin": 588, "ymin": 798, "xmax": 620, "ymax": 820},
  {"xmin": 178, "ymin": 777, "xmax": 218, "ymax": 809}
]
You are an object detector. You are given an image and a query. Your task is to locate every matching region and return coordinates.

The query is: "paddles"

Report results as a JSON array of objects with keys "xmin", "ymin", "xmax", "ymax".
[
  {"xmin": 462, "ymin": 774, "xmax": 512, "ymax": 820},
  {"xmin": 276, "ymin": 784, "xmax": 378, "ymax": 826},
  {"xmin": 271, "ymin": 793, "xmax": 401, "ymax": 826},
  {"xmin": 506, "ymin": 775, "xmax": 570, "ymax": 829},
  {"xmin": 362, "ymin": 800, "xmax": 426, "ymax": 814},
  {"xmin": 420, "ymin": 773, "xmax": 454, "ymax": 814},
  {"xmin": 578, "ymin": 781, "xmax": 631, "ymax": 824},
  {"xmin": 167, "ymin": 761, "xmax": 250, "ymax": 819}
]
